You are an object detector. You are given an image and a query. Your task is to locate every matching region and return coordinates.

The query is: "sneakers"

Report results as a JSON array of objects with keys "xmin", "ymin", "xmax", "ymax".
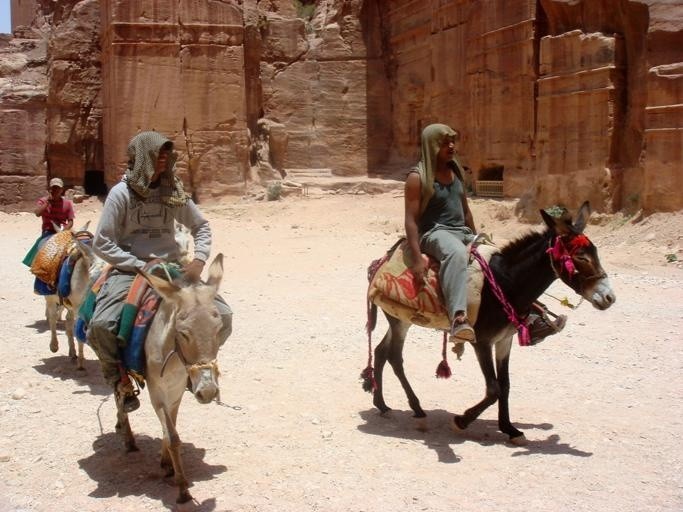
[{"xmin": 113, "ymin": 381, "xmax": 140, "ymax": 412}]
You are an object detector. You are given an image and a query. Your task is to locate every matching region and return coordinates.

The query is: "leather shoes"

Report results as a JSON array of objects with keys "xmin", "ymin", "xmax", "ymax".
[
  {"xmin": 450, "ymin": 320, "xmax": 475, "ymax": 343},
  {"xmin": 518, "ymin": 315, "xmax": 566, "ymax": 346}
]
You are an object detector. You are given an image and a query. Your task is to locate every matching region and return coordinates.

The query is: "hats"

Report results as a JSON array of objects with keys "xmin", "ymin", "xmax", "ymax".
[{"xmin": 50, "ymin": 178, "xmax": 64, "ymax": 188}]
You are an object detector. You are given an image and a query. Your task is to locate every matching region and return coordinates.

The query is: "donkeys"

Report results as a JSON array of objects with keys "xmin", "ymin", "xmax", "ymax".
[
  {"xmin": 45, "ymin": 229, "xmax": 104, "ymax": 372},
  {"xmin": 366, "ymin": 200, "xmax": 616, "ymax": 447},
  {"xmin": 87, "ymin": 254, "xmax": 224, "ymax": 511}
]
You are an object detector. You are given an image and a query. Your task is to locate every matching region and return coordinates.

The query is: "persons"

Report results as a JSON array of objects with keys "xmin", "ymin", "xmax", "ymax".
[
  {"xmin": 33, "ymin": 178, "xmax": 75, "ymax": 291},
  {"xmin": 82, "ymin": 132, "xmax": 233, "ymax": 412},
  {"xmin": 405, "ymin": 122, "xmax": 568, "ymax": 346}
]
